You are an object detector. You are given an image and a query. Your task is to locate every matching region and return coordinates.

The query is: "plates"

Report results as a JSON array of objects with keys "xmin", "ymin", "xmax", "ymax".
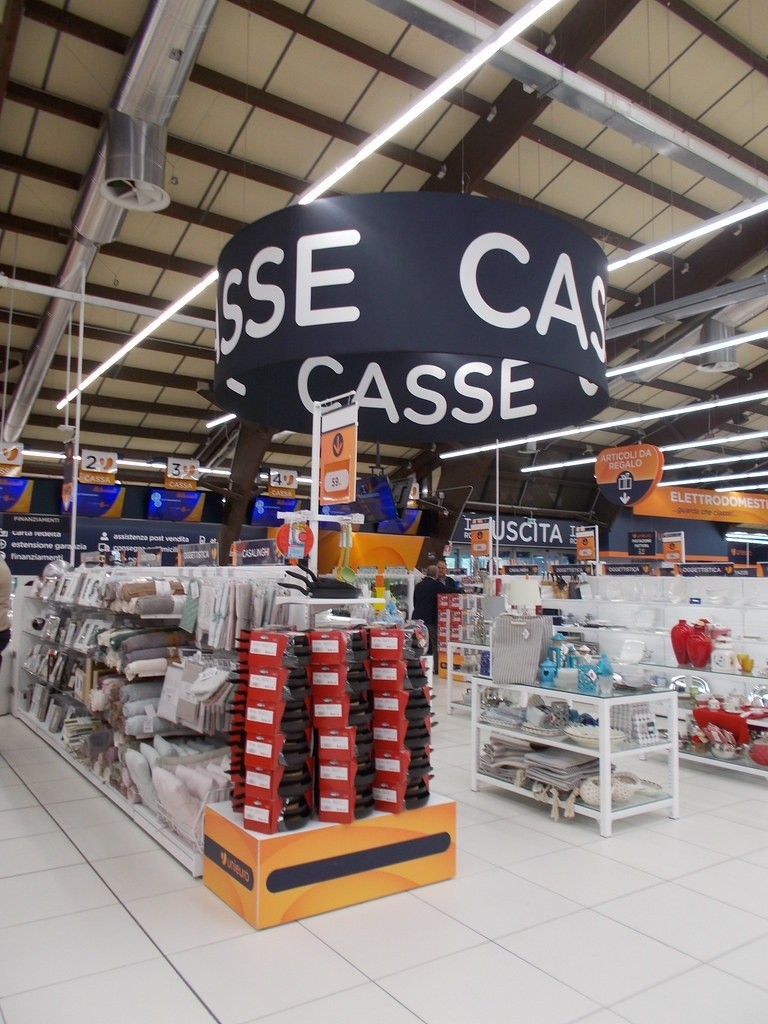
[
  {"xmin": 668, "ymin": 581, "xmax": 686, "ymax": 604},
  {"xmin": 521, "ymin": 721, "xmax": 565, "ymax": 737},
  {"xmin": 606, "ymin": 580, "xmax": 624, "ymax": 601},
  {"xmin": 620, "ymin": 640, "xmax": 645, "ymax": 664},
  {"xmin": 562, "ymin": 724, "xmax": 627, "ymax": 748}
]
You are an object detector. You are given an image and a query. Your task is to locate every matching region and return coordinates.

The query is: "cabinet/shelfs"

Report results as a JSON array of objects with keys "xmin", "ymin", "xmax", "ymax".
[
  {"xmin": 14, "ymin": 565, "xmax": 307, "ymax": 880},
  {"xmin": 474, "ymin": 668, "xmax": 680, "ymax": 837},
  {"xmin": 489, "ymin": 572, "xmax": 768, "ymax": 781}
]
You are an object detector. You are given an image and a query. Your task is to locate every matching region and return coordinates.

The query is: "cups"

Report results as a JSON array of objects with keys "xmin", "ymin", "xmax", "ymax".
[{"xmin": 736, "ymin": 654, "xmax": 754, "ymax": 675}]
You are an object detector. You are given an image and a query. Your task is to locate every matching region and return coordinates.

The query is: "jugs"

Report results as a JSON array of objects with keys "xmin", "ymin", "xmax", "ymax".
[
  {"xmin": 723, "ymin": 689, "xmax": 745, "ymax": 713},
  {"xmin": 707, "ymin": 697, "xmax": 720, "ymax": 712}
]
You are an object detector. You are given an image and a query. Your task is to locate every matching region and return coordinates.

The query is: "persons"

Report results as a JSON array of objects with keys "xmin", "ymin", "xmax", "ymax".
[
  {"xmin": 0, "ymin": 556, "xmax": 13, "ymax": 652},
  {"xmin": 411, "ymin": 560, "xmax": 466, "ymax": 673}
]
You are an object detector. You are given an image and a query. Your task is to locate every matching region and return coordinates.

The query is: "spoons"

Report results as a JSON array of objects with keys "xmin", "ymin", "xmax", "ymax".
[{"xmin": 332, "ymin": 523, "xmax": 357, "ymax": 583}]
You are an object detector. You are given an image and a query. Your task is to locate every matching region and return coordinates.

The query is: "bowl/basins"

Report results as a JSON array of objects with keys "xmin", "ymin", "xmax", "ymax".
[{"xmin": 710, "ymin": 742, "xmax": 742, "ymax": 759}]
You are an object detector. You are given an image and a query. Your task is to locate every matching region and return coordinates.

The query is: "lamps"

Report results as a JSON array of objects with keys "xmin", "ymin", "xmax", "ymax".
[
  {"xmin": 725, "ymin": 523, "xmax": 768, "ymax": 545},
  {"xmin": 22, "ymin": 0, "xmax": 768, "ymax": 496}
]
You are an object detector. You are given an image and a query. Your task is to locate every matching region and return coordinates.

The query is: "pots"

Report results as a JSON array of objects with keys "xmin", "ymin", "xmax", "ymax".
[{"xmin": 277, "ymin": 563, "xmax": 361, "ymax": 599}]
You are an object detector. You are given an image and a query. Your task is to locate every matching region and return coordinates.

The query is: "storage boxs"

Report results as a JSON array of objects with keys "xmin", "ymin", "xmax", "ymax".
[{"xmin": 438, "ymin": 592, "xmax": 471, "ymax": 684}]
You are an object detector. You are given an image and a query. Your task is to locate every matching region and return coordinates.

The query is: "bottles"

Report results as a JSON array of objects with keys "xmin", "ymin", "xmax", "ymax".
[
  {"xmin": 686, "ymin": 625, "xmax": 712, "ymax": 668},
  {"xmin": 671, "ymin": 619, "xmax": 695, "ymax": 665}
]
